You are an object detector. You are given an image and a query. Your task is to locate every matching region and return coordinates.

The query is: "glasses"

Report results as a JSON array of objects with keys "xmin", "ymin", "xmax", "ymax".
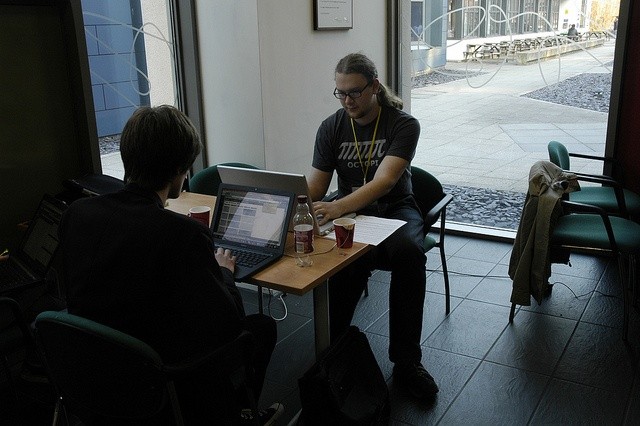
[{"xmin": 333, "ymin": 82, "xmax": 369, "ymax": 99}]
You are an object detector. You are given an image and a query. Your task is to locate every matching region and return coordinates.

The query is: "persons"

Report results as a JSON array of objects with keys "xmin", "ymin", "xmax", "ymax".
[
  {"xmin": 60, "ymin": 104, "xmax": 285, "ymax": 425},
  {"xmin": 307, "ymin": 51, "xmax": 440, "ymax": 400}
]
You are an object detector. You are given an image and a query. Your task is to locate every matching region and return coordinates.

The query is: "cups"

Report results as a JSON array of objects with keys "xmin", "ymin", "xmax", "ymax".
[
  {"xmin": 333, "ymin": 217, "xmax": 356, "ymax": 249},
  {"xmin": 187, "ymin": 206, "xmax": 211, "ymax": 228}
]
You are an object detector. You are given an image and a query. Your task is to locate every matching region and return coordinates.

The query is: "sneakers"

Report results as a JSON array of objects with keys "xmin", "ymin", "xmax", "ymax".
[
  {"xmin": 393, "ymin": 362, "xmax": 440, "ymax": 398},
  {"xmin": 239, "ymin": 401, "xmax": 285, "ymax": 426}
]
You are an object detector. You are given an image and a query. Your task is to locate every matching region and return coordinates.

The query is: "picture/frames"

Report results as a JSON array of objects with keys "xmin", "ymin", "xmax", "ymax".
[{"xmin": 313, "ymin": 0, "xmax": 353, "ymax": 31}]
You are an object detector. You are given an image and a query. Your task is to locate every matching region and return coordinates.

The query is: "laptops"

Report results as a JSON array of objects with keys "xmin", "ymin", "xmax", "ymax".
[
  {"xmin": 217, "ymin": 165, "xmax": 356, "ymax": 237},
  {"xmin": 210, "ymin": 183, "xmax": 296, "ymax": 282},
  {"xmin": 1, "ymin": 194, "xmax": 70, "ymax": 295}
]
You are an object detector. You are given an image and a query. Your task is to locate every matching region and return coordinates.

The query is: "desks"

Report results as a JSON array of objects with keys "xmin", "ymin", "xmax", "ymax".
[{"xmin": 160, "ymin": 189, "xmax": 407, "ymax": 366}]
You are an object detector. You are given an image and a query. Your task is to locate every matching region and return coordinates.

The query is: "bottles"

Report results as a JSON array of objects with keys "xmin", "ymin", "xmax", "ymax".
[{"xmin": 293, "ymin": 195, "xmax": 315, "ymax": 267}]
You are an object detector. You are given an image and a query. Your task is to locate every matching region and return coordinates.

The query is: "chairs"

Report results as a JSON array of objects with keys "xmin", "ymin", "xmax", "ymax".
[
  {"xmin": 408, "ymin": 167, "xmax": 452, "ymax": 319},
  {"xmin": 510, "ymin": 161, "xmax": 639, "ymax": 340},
  {"xmin": 0, "ymin": 297, "xmax": 54, "ymax": 408},
  {"xmin": 458, "ymin": 32, "xmax": 609, "ymax": 63},
  {"xmin": 548, "ymin": 141, "xmax": 640, "ymax": 289},
  {"xmin": 188, "ymin": 163, "xmax": 259, "ymax": 195},
  {"xmin": 28, "ymin": 311, "xmax": 260, "ymax": 424}
]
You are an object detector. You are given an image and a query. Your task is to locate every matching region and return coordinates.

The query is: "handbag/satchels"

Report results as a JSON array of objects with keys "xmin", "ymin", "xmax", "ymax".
[{"xmin": 297, "ymin": 324, "xmax": 389, "ymax": 425}]
